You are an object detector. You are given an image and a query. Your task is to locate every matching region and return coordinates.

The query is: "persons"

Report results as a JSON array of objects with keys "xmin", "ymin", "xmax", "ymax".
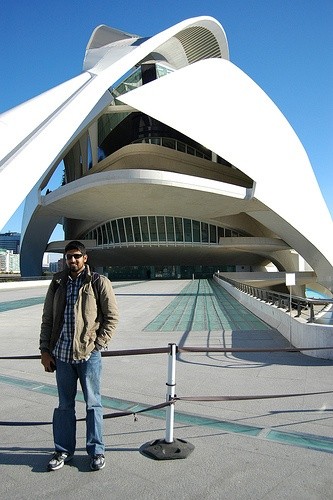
[{"xmin": 39, "ymin": 240, "xmax": 119, "ymax": 471}]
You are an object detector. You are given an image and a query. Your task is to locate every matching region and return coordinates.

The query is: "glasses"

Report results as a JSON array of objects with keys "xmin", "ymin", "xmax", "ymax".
[{"xmin": 63, "ymin": 253, "xmax": 84, "ymax": 260}]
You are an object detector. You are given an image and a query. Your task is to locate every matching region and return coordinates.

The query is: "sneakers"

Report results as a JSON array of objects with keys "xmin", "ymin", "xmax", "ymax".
[
  {"xmin": 88, "ymin": 453, "xmax": 106, "ymax": 470},
  {"xmin": 47, "ymin": 452, "xmax": 73, "ymax": 471}
]
